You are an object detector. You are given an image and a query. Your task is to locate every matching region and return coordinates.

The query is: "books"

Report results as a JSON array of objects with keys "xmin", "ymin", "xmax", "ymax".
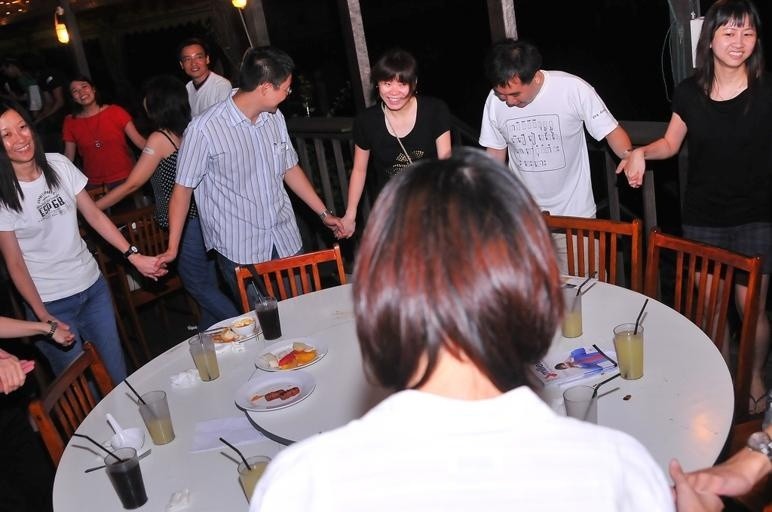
[{"xmin": 529, "ymin": 342, "xmax": 619, "ymax": 388}]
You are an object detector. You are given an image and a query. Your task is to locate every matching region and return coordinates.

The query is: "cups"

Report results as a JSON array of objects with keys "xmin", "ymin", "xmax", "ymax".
[
  {"xmin": 253, "ymin": 295, "xmax": 283, "ymax": 341},
  {"xmin": 239, "ymin": 456, "xmax": 271, "ymax": 503},
  {"xmin": 612, "ymin": 324, "xmax": 644, "ymax": 379},
  {"xmin": 189, "ymin": 334, "xmax": 221, "ymax": 382},
  {"xmin": 561, "ymin": 287, "xmax": 584, "ymax": 339},
  {"xmin": 104, "ymin": 448, "xmax": 149, "ymax": 510},
  {"xmin": 563, "ymin": 386, "xmax": 598, "ymax": 427},
  {"xmin": 140, "ymin": 391, "xmax": 176, "ymax": 445}
]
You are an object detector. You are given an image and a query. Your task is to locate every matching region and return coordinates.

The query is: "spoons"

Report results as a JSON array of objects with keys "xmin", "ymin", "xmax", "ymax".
[{"xmin": 105, "ymin": 411, "xmax": 136, "ymax": 444}]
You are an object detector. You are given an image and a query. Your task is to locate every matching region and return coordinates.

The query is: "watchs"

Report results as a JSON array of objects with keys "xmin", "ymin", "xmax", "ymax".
[
  {"xmin": 122, "ymin": 241, "xmax": 140, "ymax": 257},
  {"xmin": 743, "ymin": 431, "xmax": 771, "ymax": 464}
]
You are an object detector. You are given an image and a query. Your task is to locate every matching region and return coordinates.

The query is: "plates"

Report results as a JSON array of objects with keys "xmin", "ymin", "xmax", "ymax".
[
  {"xmin": 233, "ymin": 370, "xmax": 316, "ymax": 412},
  {"xmin": 99, "ymin": 440, "xmax": 151, "ymax": 456},
  {"xmin": 206, "ymin": 317, "xmax": 263, "ymax": 344},
  {"xmin": 253, "ymin": 338, "xmax": 329, "ymax": 371}
]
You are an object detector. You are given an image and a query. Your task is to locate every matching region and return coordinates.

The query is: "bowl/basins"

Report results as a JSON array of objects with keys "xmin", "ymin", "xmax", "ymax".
[
  {"xmin": 110, "ymin": 428, "xmax": 146, "ymax": 451},
  {"xmin": 230, "ymin": 317, "xmax": 256, "ymax": 336}
]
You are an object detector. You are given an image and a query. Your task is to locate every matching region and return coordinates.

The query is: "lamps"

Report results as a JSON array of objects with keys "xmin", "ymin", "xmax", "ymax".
[{"xmin": 54, "ymin": 5, "xmax": 70, "ymax": 44}]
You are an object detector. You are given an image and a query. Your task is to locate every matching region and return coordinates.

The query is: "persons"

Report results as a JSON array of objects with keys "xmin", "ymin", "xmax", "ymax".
[
  {"xmin": 481, "ymin": 39, "xmax": 645, "ymax": 282},
  {"xmin": 0, "ymin": 94, "xmax": 169, "ymax": 448},
  {"xmin": 95, "ymin": 73, "xmax": 242, "ymax": 333},
  {"xmin": 0, "ymin": 316, "xmax": 75, "ymax": 396},
  {"xmin": 554, "ymin": 346, "xmax": 608, "ymax": 373},
  {"xmin": 667, "ymin": 402, "xmax": 771, "ymax": 498},
  {"xmin": 1, "ymin": 53, "xmax": 81, "ymax": 166},
  {"xmin": 62, "ymin": 75, "xmax": 149, "ymax": 293},
  {"xmin": 321, "ymin": 52, "xmax": 453, "ymax": 241},
  {"xmin": 240, "ymin": 147, "xmax": 722, "ymax": 512},
  {"xmin": 624, "ymin": 0, "xmax": 771, "ymax": 414},
  {"xmin": 0, "ymin": 353, "xmax": 36, "ymax": 395},
  {"xmin": 154, "ymin": 46, "xmax": 356, "ymax": 315},
  {"xmin": 177, "ymin": 39, "xmax": 232, "ymax": 119}
]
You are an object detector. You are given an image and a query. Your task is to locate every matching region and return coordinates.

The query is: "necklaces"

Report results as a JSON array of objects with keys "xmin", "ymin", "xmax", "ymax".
[{"xmin": 79, "ymin": 104, "xmax": 103, "ymax": 149}]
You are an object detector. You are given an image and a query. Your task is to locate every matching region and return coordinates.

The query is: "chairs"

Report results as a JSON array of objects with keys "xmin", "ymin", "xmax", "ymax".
[
  {"xmin": 642, "ymin": 226, "xmax": 764, "ymax": 462},
  {"xmin": 28, "ymin": 339, "xmax": 116, "ymax": 471},
  {"xmin": 234, "ymin": 244, "xmax": 347, "ymax": 314},
  {"xmin": 538, "ymin": 210, "xmax": 644, "ymax": 293},
  {"xmin": 78, "ymin": 185, "xmax": 202, "ymax": 368}
]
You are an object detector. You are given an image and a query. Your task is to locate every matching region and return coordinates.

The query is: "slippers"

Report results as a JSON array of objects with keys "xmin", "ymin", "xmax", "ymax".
[{"xmin": 747, "ymin": 391, "xmax": 769, "ymax": 420}]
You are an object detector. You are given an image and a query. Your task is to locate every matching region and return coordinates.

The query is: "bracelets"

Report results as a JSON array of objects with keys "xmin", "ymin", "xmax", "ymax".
[
  {"xmin": 320, "ymin": 206, "xmax": 333, "ymax": 221},
  {"xmin": 47, "ymin": 322, "xmax": 58, "ymax": 338},
  {"xmin": 621, "ymin": 148, "xmax": 633, "ymax": 160}
]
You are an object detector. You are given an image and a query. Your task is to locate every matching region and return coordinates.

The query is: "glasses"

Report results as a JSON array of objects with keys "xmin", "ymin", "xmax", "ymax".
[{"xmin": 271, "ymin": 83, "xmax": 293, "ymax": 95}]
